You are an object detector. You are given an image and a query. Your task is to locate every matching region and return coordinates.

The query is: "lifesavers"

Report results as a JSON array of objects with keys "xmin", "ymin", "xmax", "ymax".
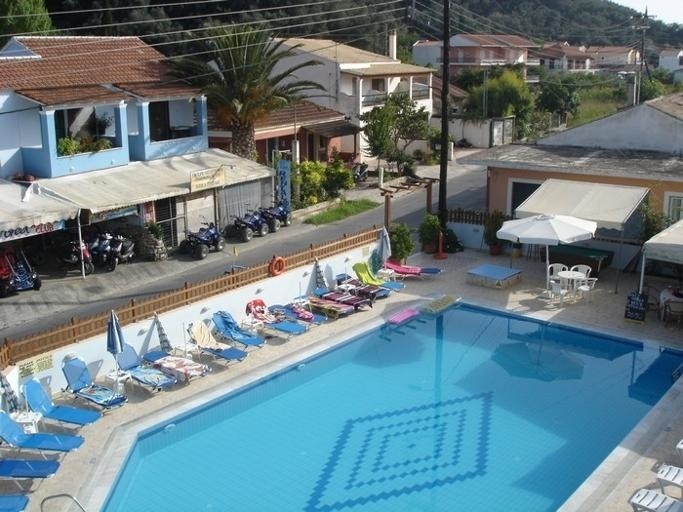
[{"xmin": 271, "ymin": 256, "xmax": 286, "ymax": 275}]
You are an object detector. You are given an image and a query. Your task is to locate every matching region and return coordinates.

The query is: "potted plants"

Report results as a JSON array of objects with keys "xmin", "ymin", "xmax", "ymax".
[
  {"xmin": 414, "ymin": 213, "xmax": 440, "ymax": 253},
  {"xmin": 387, "ymin": 221, "xmax": 413, "ymax": 265},
  {"xmin": 480, "ymin": 209, "xmax": 503, "ymax": 258}
]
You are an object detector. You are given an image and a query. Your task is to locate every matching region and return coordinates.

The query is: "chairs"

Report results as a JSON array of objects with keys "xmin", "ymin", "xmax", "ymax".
[
  {"xmin": 1, "ymin": 494, "xmax": 30, "ymax": 511},
  {"xmin": 19, "ymin": 376, "xmax": 101, "ymax": 428},
  {"xmin": 60, "ymin": 352, "xmax": 129, "ymax": 417},
  {"xmin": 107, "ymin": 343, "xmax": 179, "ymax": 396},
  {"xmin": 185, "ymin": 259, "xmax": 444, "ymax": 371},
  {"xmin": 545, "ymin": 262, "xmax": 598, "ymax": 310},
  {"xmin": 628, "ymin": 489, "xmax": 682, "ymax": 511},
  {"xmin": 656, "ymin": 464, "xmax": 682, "ymax": 495},
  {"xmin": 143, "ymin": 350, "xmax": 210, "ymax": 385},
  {"xmin": 637, "ymin": 277, "xmax": 683, "ymax": 329},
  {"xmin": 675, "ymin": 439, "xmax": 682, "ymax": 455},
  {"xmin": 0, "ymin": 410, "xmax": 85, "ymax": 462},
  {"xmin": 0, "ymin": 456, "xmax": 60, "ymax": 492}
]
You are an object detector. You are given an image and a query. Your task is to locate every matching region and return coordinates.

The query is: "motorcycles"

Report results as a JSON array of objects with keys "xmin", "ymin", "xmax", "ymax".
[
  {"xmin": 225, "ymin": 206, "xmax": 269, "ymax": 242},
  {"xmin": 86, "ymin": 230, "xmax": 136, "ymax": 272},
  {"xmin": 56, "ymin": 237, "xmax": 94, "ymax": 275},
  {"xmin": 178, "ymin": 215, "xmax": 225, "ymax": 259},
  {"xmin": 352, "ymin": 163, "xmax": 368, "ymax": 183},
  {"xmin": 258, "ymin": 191, "xmax": 293, "ymax": 232},
  {"xmin": 0, "ymin": 239, "xmax": 40, "ymax": 298}
]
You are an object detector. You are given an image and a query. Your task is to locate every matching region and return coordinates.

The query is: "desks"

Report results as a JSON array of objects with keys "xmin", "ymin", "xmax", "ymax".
[
  {"xmin": 464, "ymin": 263, "xmax": 525, "ymax": 289},
  {"xmin": 539, "ymin": 244, "xmax": 615, "ymax": 280}
]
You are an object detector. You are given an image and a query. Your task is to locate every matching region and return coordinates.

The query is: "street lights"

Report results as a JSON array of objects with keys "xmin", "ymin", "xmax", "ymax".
[{"xmin": 617, "ymin": 71, "xmax": 636, "ymax": 107}]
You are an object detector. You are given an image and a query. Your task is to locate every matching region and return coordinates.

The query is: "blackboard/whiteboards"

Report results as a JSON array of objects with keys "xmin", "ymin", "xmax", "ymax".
[{"xmin": 623, "ymin": 292, "xmax": 648, "ymax": 321}]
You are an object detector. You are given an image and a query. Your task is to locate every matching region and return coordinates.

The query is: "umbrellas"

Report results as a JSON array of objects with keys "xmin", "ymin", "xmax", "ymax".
[
  {"xmin": 151, "ymin": 310, "xmax": 172, "ymax": 354},
  {"xmin": 495, "ymin": 213, "xmax": 598, "ymax": 288},
  {"xmin": 376, "ymin": 225, "xmax": 392, "ymax": 271},
  {"xmin": 0, "ymin": 373, "xmax": 22, "ymax": 413},
  {"xmin": 106, "ymin": 308, "xmax": 124, "ymax": 372},
  {"xmin": 314, "ymin": 255, "xmax": 329, "ymax": 288}
]
